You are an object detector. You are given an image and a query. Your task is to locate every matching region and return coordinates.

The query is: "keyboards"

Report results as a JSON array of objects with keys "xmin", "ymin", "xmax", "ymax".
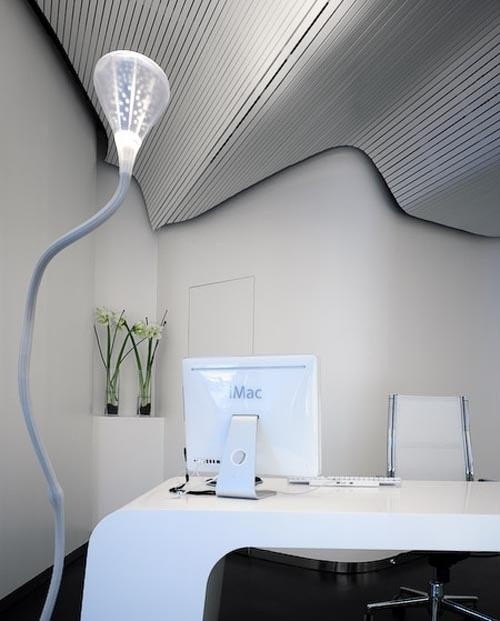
[{"xmin": 287, "ymin": 478, "xmax": 398, "ymax": 487}]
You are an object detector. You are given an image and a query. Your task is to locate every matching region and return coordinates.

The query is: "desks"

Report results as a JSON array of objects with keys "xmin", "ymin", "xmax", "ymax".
[{"xmin": 77, "ymin": 474, "xmax": 500, "ymax": 621}]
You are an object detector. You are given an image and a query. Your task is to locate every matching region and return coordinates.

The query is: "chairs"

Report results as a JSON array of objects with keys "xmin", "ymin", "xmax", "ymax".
[{"xmin": 365, "ymin": 393, "xmax": 500, "ymax": 620}]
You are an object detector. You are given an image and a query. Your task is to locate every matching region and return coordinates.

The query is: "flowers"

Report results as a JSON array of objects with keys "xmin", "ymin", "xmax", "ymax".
[
  {"xmin": 91, "ymin": 305, "xmax": 155, "ymax": 399},
  {"xmin": 120, "ymin": 309, "xmax": 170, "ymax": 397}
]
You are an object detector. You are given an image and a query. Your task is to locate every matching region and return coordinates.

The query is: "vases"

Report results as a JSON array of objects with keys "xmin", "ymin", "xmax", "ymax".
[
  {"xmin": 135, "ymin": 369, "xmax": 152, "ymax": 415},
  {"xmin": 105, "ymin": 366, "xmax": 121, "ymax": 414}
]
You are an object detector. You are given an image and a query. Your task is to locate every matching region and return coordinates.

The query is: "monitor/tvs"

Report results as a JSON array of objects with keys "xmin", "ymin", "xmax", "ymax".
[{"xmin": 179, "ymin": 352, "xmax": 321, "ymax": 501}]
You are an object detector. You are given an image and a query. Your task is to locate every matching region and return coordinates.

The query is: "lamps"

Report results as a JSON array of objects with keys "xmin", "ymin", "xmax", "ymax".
[{"xmin": 17, "ymin": 49, "xmax": 171, "ymax": 621}]
